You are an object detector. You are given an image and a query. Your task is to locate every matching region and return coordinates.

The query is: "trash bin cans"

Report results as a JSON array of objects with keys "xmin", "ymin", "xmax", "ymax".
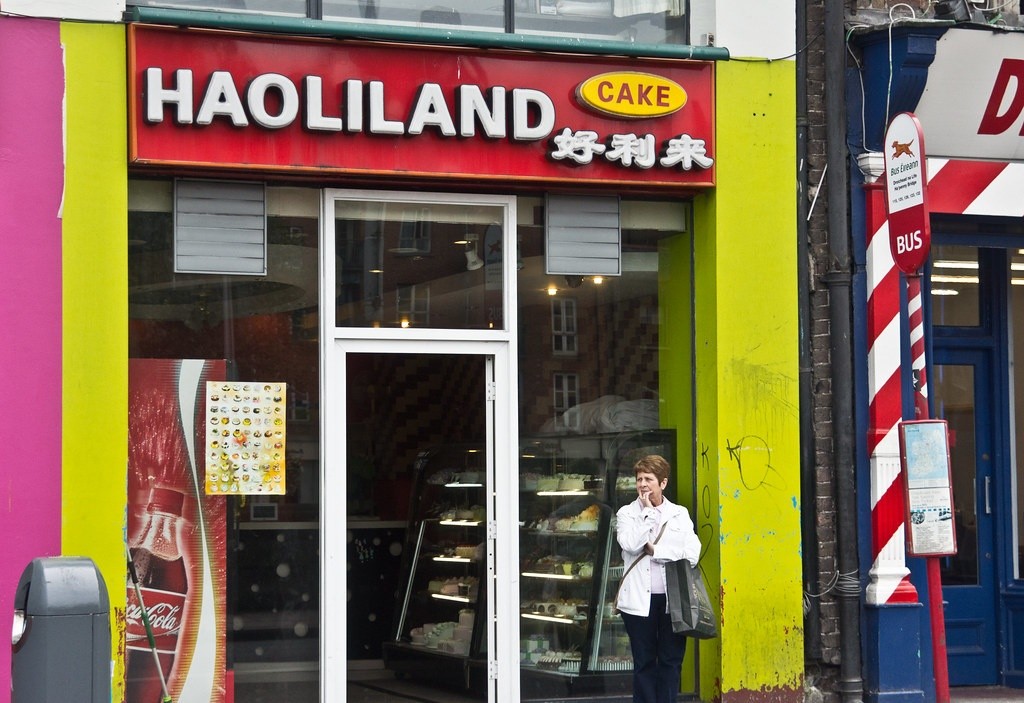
[{"xmin": 12, "ymin": 555, "xmax": 112, "ymax": 703}]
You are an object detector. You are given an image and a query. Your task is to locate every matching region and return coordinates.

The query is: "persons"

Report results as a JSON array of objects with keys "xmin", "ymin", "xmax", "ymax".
[{"xmin": 615, "ymin": 455, "xmax": 701, "ymax": 703}]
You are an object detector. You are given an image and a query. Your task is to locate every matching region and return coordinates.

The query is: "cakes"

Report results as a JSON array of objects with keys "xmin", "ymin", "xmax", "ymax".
[{"xmin": 410, "ymin": 472, "xmax": 636, "ymax": 672}]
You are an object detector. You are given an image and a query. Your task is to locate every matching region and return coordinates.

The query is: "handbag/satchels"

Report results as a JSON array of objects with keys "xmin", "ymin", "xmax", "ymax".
[
  {"xmin": 665, "ymin": 559, "xmax": 717, "ymax": 639},
  {"xmin": 612, "ymin": 577, "xmax": 625, "ymax": 616}
]
